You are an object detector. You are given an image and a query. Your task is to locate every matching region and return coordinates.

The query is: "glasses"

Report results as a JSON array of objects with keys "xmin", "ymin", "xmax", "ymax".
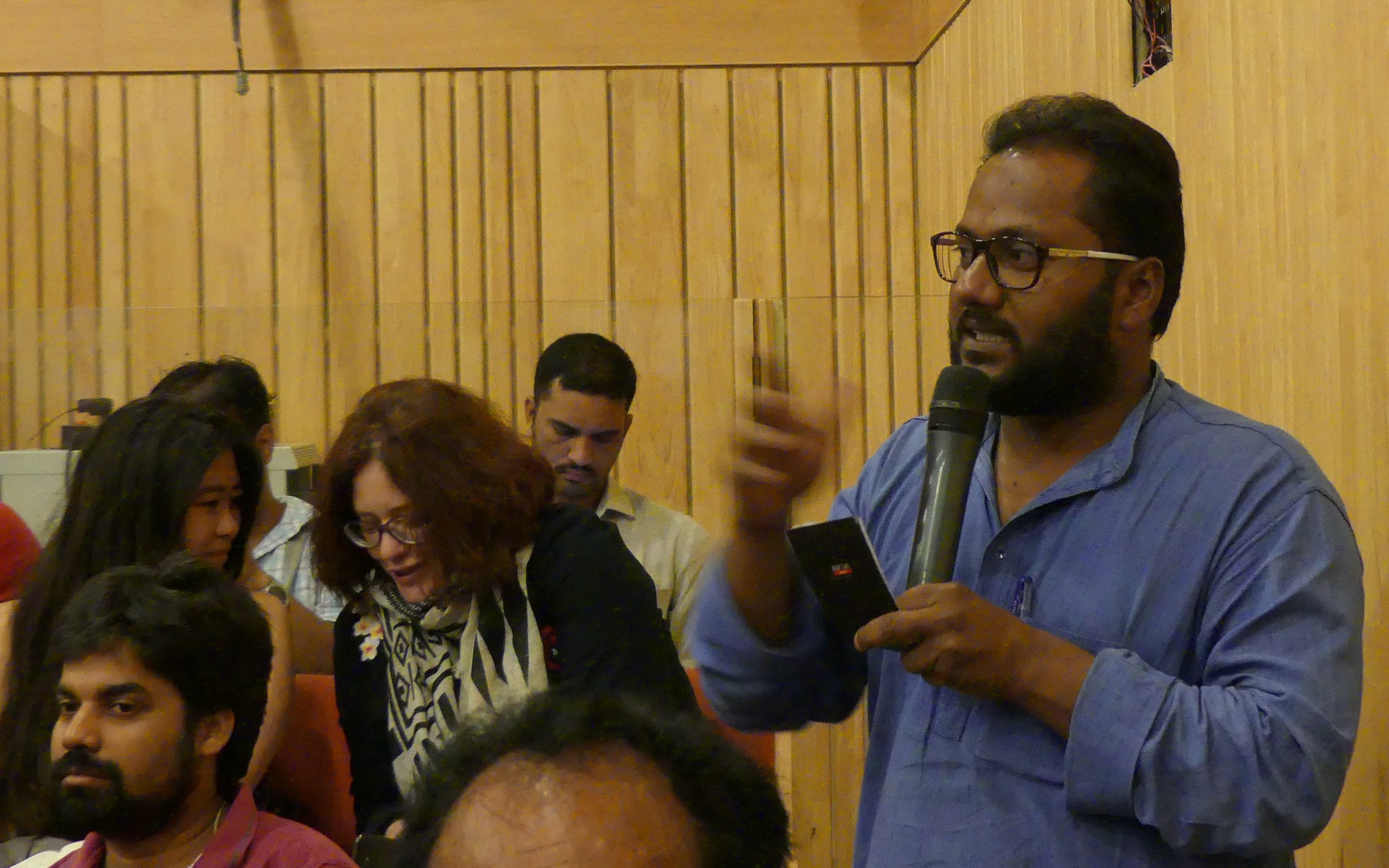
[
  {"xmin": 931, "ymin": 231, "xmax": 1141, "ymax": 291},
  {"xmin": 343, "ymin": 515, "xmax": 426, "ymax": 549}
]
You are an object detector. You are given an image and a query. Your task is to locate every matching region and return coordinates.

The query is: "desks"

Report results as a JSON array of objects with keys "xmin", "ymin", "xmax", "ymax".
[{"xmin": 0, "ymin": 443, "xmax": 323, "ymax": 548}]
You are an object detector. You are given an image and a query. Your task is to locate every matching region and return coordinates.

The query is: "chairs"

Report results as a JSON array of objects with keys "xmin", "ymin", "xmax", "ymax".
[{"xmin": 256, "ymin": 666, "xmax": 776, "ymax": 858}]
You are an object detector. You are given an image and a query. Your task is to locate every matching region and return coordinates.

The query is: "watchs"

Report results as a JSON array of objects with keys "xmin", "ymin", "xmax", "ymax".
[{"xmin": 261, "ymin": 578, "xmax": 290, "ymax": 610}]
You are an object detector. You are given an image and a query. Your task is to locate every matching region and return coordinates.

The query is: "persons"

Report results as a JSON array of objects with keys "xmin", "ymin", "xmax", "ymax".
[
  {"xmin": 525, "ymin": 332, "xmax": 711, "ymax": 668},
  {"xmin": 0, "ymin": 551, "xmax": 359, "ymax": 868},
  {"xmin": 678, "ymin": 91, "xmax": 1367, "ymax": 868},
  {"xmin": 0, "ymin": 356, "xmax": 373, "ymax": 802},
  {"xmin": 311, "ymin": 379, "xmax": 703, "ymax": 838},
  {"xmin": 355, "ymin": 684, "xmax": 792, "ymax": 868}
]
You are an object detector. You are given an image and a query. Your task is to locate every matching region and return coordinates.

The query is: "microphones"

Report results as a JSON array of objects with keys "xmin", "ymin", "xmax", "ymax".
[{"xmin": 907, "ymin": 365, "xmax": 993, "ymax": 590}]
